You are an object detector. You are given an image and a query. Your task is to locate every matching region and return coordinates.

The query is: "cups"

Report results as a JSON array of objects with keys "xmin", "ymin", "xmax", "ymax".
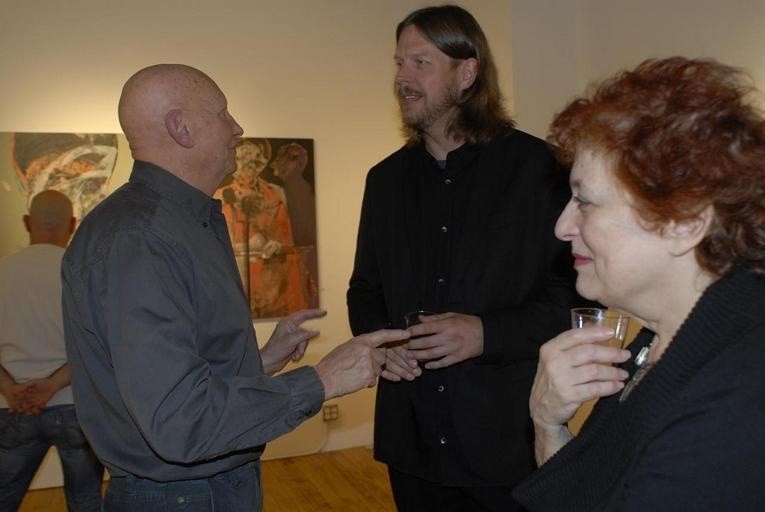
[
  {"xmin": 570, "ymin": 307, "xmax": 631, "ymax": 352},
  {"xmin": 404, "ymin": 310, "xmax": 441, "ymax": 328}
]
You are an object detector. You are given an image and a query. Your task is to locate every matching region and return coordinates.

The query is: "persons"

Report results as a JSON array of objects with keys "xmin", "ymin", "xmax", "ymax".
[
  {"xmin": 501, "ymin": 53, "xmax": 764, "ymax": 510},
  {"xmin": 0, "ymin": 188, "xmax": 106, "ymax": 511},
  {"xmin": 59, "ymin": 64, "xmax": 413, "ymax": 511},
  {"xmin": 342, "ymin": 4, "xmax": 608, "ymax": 511}
]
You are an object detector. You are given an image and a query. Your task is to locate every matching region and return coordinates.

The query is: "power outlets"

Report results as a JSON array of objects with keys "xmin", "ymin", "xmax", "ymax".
[{"xmin": 322, "ymin": 403, "xmax": 339, "ymax": 422}]
